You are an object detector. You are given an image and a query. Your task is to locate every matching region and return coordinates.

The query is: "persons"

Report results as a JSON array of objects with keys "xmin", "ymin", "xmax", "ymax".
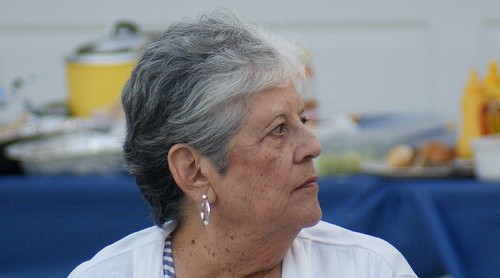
[{"xmin": 65, "ymin": 8, "xmax": 419, "ymax": 278}]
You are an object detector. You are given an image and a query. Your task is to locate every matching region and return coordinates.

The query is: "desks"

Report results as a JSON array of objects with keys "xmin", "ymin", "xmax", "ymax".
[{"xmin": 0, "ymin": 144, "xmax": 500, "ymax": 278}]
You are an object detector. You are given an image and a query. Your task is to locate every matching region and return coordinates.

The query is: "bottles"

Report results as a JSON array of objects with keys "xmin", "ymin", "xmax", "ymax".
[
  {"xmin": 480, "ymin": 60, "xmax": 500, "ymax": 136},
  {"xmin": 456, "ymin": 70, "xmax": 489, "ymax": 158}
]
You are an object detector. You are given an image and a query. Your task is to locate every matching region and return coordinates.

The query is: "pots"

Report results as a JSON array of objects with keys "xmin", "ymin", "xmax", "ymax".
[{"xmin": 66, "ymin": 18, "xmax": 160, "ymax": 120}]
